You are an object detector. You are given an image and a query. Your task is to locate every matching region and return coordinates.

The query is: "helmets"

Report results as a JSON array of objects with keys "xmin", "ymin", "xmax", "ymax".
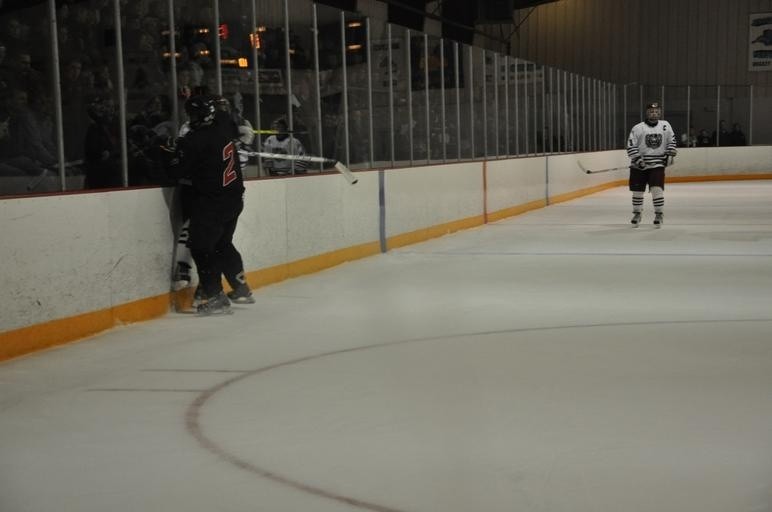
[
  {"xmin": 184, "ymin": 95, "xmax": 216, "ymax": 128},
  {"xmin": 646, "ymin": 102, "xmax": 662, "ymax": 120}
]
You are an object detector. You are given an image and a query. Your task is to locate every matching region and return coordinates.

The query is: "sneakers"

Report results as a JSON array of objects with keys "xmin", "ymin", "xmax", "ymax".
[
  {"xmin": 228, "ymin": 285, "xmax": 253, "ymax": 298},
  {"xmin": 197, "ymin": 292, "xmax": 230, "ymax": 312},
  {"xmin": 655, "ymin": 213, "xmax": 663, "ymax": 224},
  {"xmin": 632, "ymin": 214, "xmax": 641, "ymax": 223}
]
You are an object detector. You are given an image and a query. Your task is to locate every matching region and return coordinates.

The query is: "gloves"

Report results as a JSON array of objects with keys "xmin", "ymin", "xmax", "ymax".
[
  {"xmin": 635, "ymin": 159, "xmax": 646, "ymax": 168},
  {"xmin": 664, "ymin": 156, "xmax": 673, "ymax": 166}
]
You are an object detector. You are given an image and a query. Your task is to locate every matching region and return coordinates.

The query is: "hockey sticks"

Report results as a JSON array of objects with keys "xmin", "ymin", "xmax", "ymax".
[
  {"xmin": 238, "ymin": 150, "xmax": 358, "ymax": 185},
  {"xmin": 577, "ymin": 160, "xmax": 667, "ymax": 174}
]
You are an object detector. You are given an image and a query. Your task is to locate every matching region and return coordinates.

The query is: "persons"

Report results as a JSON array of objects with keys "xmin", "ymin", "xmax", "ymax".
[
  {"xmin": 147, "ymin": 96, "xmax": 255, "ymax": 311},
  {"xmin": 410, "ymin": 34, "xmax": 452, "ymax": 91},
  {"xmin": 626, "ymin": 101, "xmax": 677, "ymax": 225},
  {"xmin": 319, "ymin": 38, "xmax": 355, "ymax": 104},
  {"xmin": 168, "ymin": 95, "xmax": 256, "ymax": 312},
  {"xmin": 675, "ymin": 119, "xmax": 746, "ymax": 146},
  {"xmin": 1, "ymin": 3, "xmax": 308, "ymax": 188},
  {"xmin": 489, "ymin": 39, "xmax": 510, "ymax": 81}
]
